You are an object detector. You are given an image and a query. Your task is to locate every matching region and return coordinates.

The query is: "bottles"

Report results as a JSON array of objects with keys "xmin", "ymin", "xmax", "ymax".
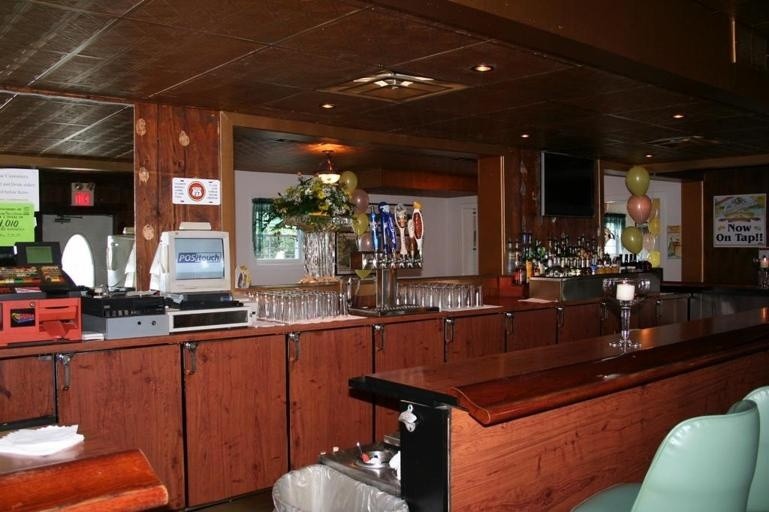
[{"xmin": 507, "ymin": 232, "xmax": 638, "ymax": 286}]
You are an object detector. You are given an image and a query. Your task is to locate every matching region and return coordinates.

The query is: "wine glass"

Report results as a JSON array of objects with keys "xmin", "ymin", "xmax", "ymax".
[{"xmin": 601, "ymin": 277, "xmax": 651, "ymax": 347}]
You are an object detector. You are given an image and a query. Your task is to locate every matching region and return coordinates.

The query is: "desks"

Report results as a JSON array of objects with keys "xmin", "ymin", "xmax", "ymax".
[
  {"xmin": 0, "ymin": 424, "xmax": 169, "ymax": 511},
  {"xmin": 314, "ymin": 306, "xmax": 769, "ymax": 512}
]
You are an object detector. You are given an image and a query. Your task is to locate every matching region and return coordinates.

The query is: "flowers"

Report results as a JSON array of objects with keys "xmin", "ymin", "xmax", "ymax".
[{"xmin": 262, "ymin": 177, "xmax": 355, "ymax": 232}]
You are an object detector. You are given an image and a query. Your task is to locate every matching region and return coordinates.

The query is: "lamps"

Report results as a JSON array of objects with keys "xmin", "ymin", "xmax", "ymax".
[{"xmin": 316, "ymin": 149, "xmax": 340, "ymax": 183}]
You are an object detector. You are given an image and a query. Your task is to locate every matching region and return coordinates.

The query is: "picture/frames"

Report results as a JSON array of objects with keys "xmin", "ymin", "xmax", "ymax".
[{"xmin": 335, "ymin": 231, "xmax": 376, "ymax": 276}]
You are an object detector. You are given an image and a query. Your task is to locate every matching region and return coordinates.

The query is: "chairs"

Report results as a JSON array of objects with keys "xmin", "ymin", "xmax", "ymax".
[{"xmin": 569, "ymin": 386, "xmax": 769, "ymax": 512}]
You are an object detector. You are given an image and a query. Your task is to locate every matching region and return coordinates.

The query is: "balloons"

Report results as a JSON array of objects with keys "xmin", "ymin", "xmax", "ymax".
[
  {"xmin": 352, "ymin": 189, "xmax": 369, "ymax": 215},
  {"xmin": 359, "ymin": 234, "xmax": 373, "ymax": 254},
  {"xmin": 621, "ymin": 227, "xmax": 643, "ymax": 253},
  {"xmin": 351, "ymin": 212, "xmax": 368, "ymax": 234},
  {"xmin": 646, "ymin": 198, "xmax": 661, "ymax": 267},
  {"xmin": 339, "ymin": 170, "xmax": 357, "ymax": 195},
  {"xmin": 627, "ymin": 196, "xmax": 652, "ymax": 224},
  {"xmin": 625, "ymin": 166, "xmax": 650, "ymax": 196}
]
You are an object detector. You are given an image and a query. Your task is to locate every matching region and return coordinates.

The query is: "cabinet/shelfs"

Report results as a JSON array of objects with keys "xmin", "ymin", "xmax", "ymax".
[
  {"xmin": 54, "ymin": 343, "xmax": 185, "ymax": 512},
  {"xmin": 557, "ymin": 292, "xmax": 692, "ymax": 344},
  {"xmin": 182, "ymin": 334, "xmax": 287, "ymax": 508},
  {"xmin": 287, "ymin": 327, "xmax": 374, "ymax": 470},
  {"xmin": 445, "ymin": 314, "xmax": 507, "ymax": 361},
  {"xmin": 506, "ymin": 307, "xmax": 557, "ymax": 352},
  {"xmin": 374, "ymin": 319, "xmax": 445, "ymax": 441},
  {"xmin": 0, "ymin": 354, "xmax": 56, "ymax": 424}
]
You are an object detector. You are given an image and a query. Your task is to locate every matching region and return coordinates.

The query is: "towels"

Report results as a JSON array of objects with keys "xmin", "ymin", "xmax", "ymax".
[{"xmin": 0, "ymin": 424, "xmax": 85, "ymax": 474}]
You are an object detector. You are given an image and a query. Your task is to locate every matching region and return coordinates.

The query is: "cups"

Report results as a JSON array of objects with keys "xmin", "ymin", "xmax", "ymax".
[
  {"xmin": 397, "ymin": 282, "xmax": 484, "ymax": 310},
  {"xmin": 246, "ymin": 288, "xmax": 347, "ymax": 324},
  {"xmin": 757, "ymin": 257, "xmax": 769, "ymax": 289}
]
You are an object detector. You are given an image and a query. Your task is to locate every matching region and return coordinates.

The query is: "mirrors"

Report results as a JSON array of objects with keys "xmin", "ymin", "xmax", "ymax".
[
  {"xmin": 597, "ymin": 160, "xmax": 704, "ymax": 283},
  {"xmin": 220, "ymin": 110, "xmax": 506, "ymax": 288},
  {"xmin": 0, "ymin": 92, "xmax": 138, "ymax": 298}
]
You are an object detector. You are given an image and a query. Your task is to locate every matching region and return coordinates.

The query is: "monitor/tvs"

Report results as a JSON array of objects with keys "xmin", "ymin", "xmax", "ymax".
[
  {"xmin": 540, "ymin": 150, "xmax": 595, "ymax": 217},
  {"xmin": 149, "ymin": 231, "xmax": 231, "ymax": 302}
]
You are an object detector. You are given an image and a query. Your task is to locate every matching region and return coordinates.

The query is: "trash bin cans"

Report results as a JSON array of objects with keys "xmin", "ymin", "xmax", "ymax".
[{"xmin": 271, "ymin": 463, "xmax": 409, "ymax": 512}]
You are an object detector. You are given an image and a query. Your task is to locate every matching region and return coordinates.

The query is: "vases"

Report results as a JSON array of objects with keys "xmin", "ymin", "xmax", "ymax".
[{"xmin": 305, "ymin": 230, "xmax": 335, "ymax": 279}]
